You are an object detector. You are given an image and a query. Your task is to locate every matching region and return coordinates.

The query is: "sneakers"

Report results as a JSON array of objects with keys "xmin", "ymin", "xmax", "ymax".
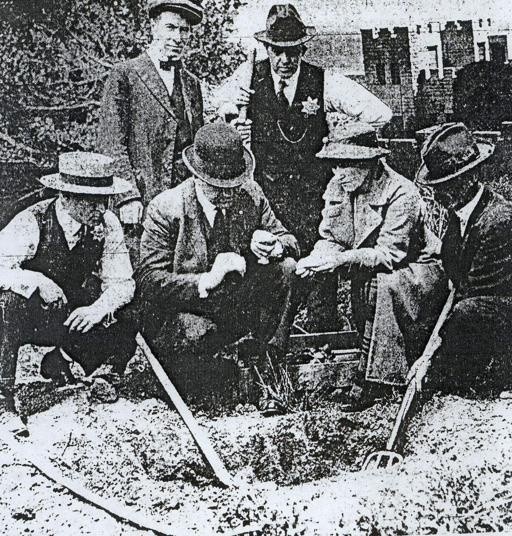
[
  {"xmin": 339, "ymin": 375, "xmax": 380, "ymax": 412},
  {"xmin": 0, "ymin": 411, "xmax": 30, "ymax": 437},
  {"xmin": 40, "ymin": 349, "xmax": 78, "ymax": 395},
  {"xmin": 258, "ymin": 394, "xmax": 288, "ymax": 418}
]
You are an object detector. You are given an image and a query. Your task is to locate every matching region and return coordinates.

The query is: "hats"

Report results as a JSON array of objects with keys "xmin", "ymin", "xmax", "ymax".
[
  {"xmin": 182, "ymin": 122, "xmax": 257, "ymax": 188},
  {"xmin": 147, "ymin": 0, "xmax": 204, "ymax": 26},
  {"xmin": 415, "ymin": 122, "xmax": 495, "ymax": 186},
  {"xmin": 254, "ymin": 3, "xmax": 317, "ymax": 48},
  {"xmin": 315, "ymin": 122, "xmax": 392, "ymax": 160},
  {"xmin": 38, "ymin": 150, "xmax": 134, "ymax": 196}
]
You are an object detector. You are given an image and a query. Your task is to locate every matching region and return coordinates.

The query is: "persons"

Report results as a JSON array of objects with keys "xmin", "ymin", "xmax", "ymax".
[
  {"xmin": 415, "ymin": 121, "xmax": 512, "ymax": 401},
  {"xmin": 0, "ymin": 151, "xmax": 136, "ymax": 438},
  {"xmin": 140, "ymin": 123, "xmax": 300, "ymax": 414},
  {"xmin": 295, "ymin": 124, "xmax": 442, "ymax": 413},
  {"xmin": 95, "ymin": 0, "xmax": 205, "ymax": 318},
  {"xmin": 211, "ymin": 4, "xmax": 393, "ymax": 306}
]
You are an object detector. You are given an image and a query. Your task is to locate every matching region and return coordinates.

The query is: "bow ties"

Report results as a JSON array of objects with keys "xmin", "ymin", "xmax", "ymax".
[{"xmin": 159, "ymin": 59, "xmax": 183, "ymax": 71}]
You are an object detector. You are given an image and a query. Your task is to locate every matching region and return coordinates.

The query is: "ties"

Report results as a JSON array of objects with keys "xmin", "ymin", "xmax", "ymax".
[
  {"xmin": 278, "ymin": 81, "xmax": 290, "ymax": 108},
  {"xmin": 214, "ymin": 206, "xmax": 228, "ymax": 252}
]
